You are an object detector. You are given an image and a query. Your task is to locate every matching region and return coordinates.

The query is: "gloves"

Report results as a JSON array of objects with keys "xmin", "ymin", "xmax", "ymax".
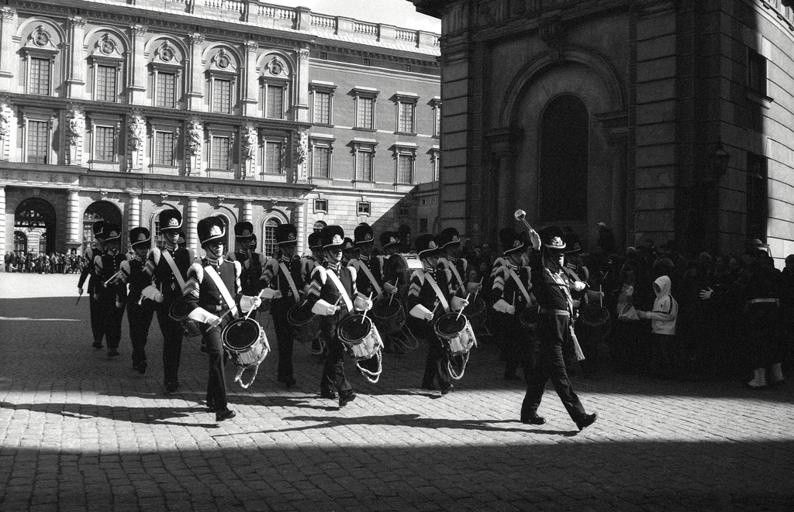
[
  {"xmin": 460, "ymin": 298, "xmax": 470, "ymax": 308},
  {"xmin": 364, "ymin": 298, "xmax": 373, "ymax": 311},
  {"xmin": 154, "ymin": 292, "xmax": 165, "ymax": 304},
  {"xmin": 251, "ymin": 295, "xmax": 262, "ymax": 309},
  {"xmin": 507, "ymin": 303, "xmax": 515, "ymax": 315},
  {"xmin": 326, "ymin": 303, "xmax": 341, "ymax": 317},
  {"xmin": 208, "ymin": 314, "xmax": 223, "ymax": 329},
  {"xmin": 273, "ymin": 288, "xmax": 283, "ymax": 299},
  {"xmin": 475, "ymin": 281, "xmax": 483, "ymax": 292},
  {"xmin": 426, "ymin": 310, "xmax": 435, "ymax": 321},
  {"xmin": 391, "ymin": 285, "xmax": 399, "ymax": 294}
]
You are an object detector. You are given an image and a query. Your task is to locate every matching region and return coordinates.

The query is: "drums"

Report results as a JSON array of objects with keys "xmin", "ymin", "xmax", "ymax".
[
  {"xmin": 520, "ymin": 305, "xmax": 542, "ymax": 342},
  {"xmin": 434, "ymin": 312, "xmax": 477, "ymax": 357},
  {"xmin": 372, "ymin": 297, "xmax": 406, "ymax": 334},
  {"xmin": 221, "ymin": 317, "xmax": 271, "ymax": 369},
  {"xmin": 454, "ymin": 295, "xmax": 487, "ymax": 328},
  {"xmin": 336, "ymin": 315, "xmax": 384, "ymax": 361},
  {"xmin": 287, "ymin": 301, "xmax": 322, "ymax": 342},
  {"xmin": 580, "ymin": 303, "xmax": 612, "ymax": 336},
  {"xmin": 384, "ymin": 253, "xmax": 425, "ymax": 301},
  {"xmin": 168, "ymin": 296, "xmax": 202, "ymax": 337}
]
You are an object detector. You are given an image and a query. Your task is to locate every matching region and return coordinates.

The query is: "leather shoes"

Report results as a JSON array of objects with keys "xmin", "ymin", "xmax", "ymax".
[
  {"xmin": 161, "ymin": 379, "xmax": 182, "ymax": 393},
  {"xmin": 319, "ymin": 387, "xmax": 357, "ymax": 407},
  {"xmin": 276, "ymin": 373, "xmax": 298, "ymax": 388},
  {"xmin": 130, "ymin": 350, "xmax": 149, "ymax": 373},
  {"xmin": 206, "ymin": 402, "xmax": 236, "ymax": 422},
  {"xmin": 575, "ymin": 411, "xmax": 597, "ymax": 431},
  {"xmin": 92, "ymin": 339, "xmax": 120, "ymax": 357},
  {"xmin": 520, "ymin": 409, "xmax": 546, "ymax": 424},
  {"xmin": 421, "ymin": 377, "xmax": 452, "ymax": 394}
]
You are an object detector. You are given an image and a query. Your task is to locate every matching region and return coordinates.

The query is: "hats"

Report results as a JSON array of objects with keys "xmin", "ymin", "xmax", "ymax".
[
  {"xmin": 197, "ymin": 215, "xmax": 227, "ymax": 249},
  {"xmin": 158, "ymin": 209, "xmax": 184, "ymax": 232},
  {"xmin": 500, "ymin": 225, "xmax": 582, "ymax": 255},
  {"xmin": 234, "ymin": 221, "xmax": 257, "ymax": 248},
  {"xmin": 92, "ymin": 220, "xmax": 123, "ymax": 243},
  {"xmin": 307, "ymin": 220, "xmax": 462, "ymax": 260},
  {"xmin": 273, "ymin": 224, "xmax": 298, "ymax": 246},
  {"xmin": 129, "ymin": 228, "xmax": 152, "ymax": 249}
]
